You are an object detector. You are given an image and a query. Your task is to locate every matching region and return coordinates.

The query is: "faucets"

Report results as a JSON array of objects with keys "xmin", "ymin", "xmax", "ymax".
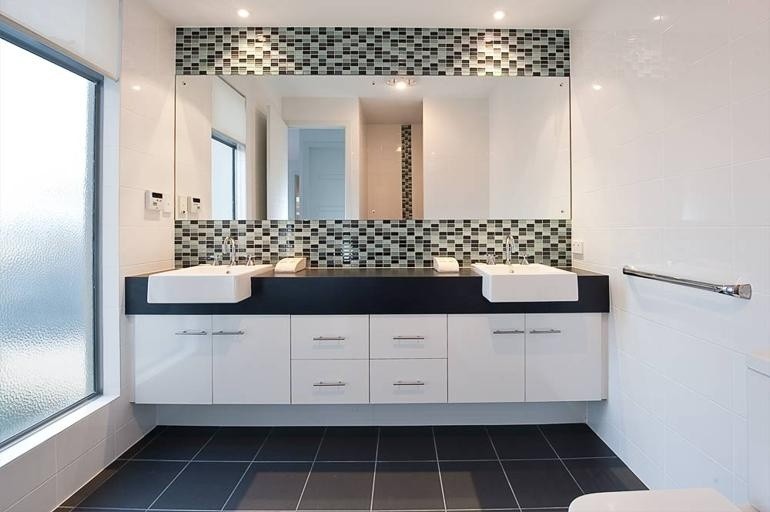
[
  {"xmin": 504, "ymin": 235, "xmax": 516, "ymax": 265},
  {"xmin": 222, "ymin": 237, "xmax": 239, "ymax": 265}
]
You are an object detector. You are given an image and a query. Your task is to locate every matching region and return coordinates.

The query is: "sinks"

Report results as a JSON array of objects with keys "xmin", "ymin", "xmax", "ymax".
[
  {"xmin": 147, "ymin": 263, "xmax": 274, "ymax": 305},
  {"xmin": 471, "ymin": 263, "xmax": 579, "ymax": 304}
]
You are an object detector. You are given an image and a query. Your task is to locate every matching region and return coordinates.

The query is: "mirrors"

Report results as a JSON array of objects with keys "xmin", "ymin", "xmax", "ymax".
[{"xmin": 176, "ymin": 73, "xmax": 572, "ymax": 224}]
